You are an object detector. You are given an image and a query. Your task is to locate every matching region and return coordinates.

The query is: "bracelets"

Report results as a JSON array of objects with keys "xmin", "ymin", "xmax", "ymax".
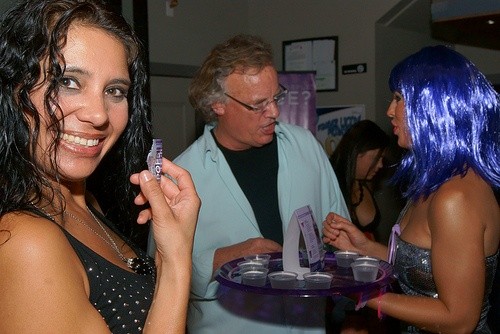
[{"xmin": 377, "ymin": 288, "xmax": 386, "ymax": 319}]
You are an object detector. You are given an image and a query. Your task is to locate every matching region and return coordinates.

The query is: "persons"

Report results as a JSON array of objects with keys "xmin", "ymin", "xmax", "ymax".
[
  {"xmin": 330, "ymin": 120, "xmax": 391, "ymax": 334},
  {"xmin": 322, "ymin": 46, "xmax": 500, "ymax": 334},
  {"xmin": 0, "ymin": 0, "xmax": 204, "ymax": 334},
  {"xmin": 147, "ymin": 33, "xmax": 354, "ymax": 334}
]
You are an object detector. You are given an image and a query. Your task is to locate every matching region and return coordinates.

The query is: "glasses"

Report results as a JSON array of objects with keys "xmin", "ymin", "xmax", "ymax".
[{"xmin": 224, "ymin": 83, "xmax": 288, "ymax": 114}]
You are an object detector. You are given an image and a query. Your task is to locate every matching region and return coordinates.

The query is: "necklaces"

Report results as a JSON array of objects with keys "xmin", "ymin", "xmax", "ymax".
[
  {"xmin": 37, "ymin": 194, "xmax": 154, "ymax": 276},
  {"xmin": 353, "ymin": 180, "xmax": 365, "ymax": 208},
  {"xmin": 386, "ymin": 194, "xmax": 415, "ymax": 265}
]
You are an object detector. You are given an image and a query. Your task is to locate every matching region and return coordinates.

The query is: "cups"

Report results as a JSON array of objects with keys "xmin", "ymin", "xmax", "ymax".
[
  {"xmin": 237, "ymin": 261, "xmax": 267, "ymax": 273},
  {"xmin": 243, "ymin": 254, "xmax": 270, "ymax": 269},
  {"xmin": 334, "ymin": 249, "xmax": 359, "ymax": 267},
  {"xmin": 353, "ymin": 256, "xmax": 381, "ymax": 266},
  {"xmin": 238, "ymin": 269, "xmax": 269, "ymax": 286},
  {"xmin": 302, "ymin": 272, "xmax": 334, "ymax": 290},
  {"xmin": 268, "ymin": 271, "xmax": 298, "ymax": 288},
  {"xmin": 351, "ymin": 262, "xmax": 380, "ymax": 282}
]
